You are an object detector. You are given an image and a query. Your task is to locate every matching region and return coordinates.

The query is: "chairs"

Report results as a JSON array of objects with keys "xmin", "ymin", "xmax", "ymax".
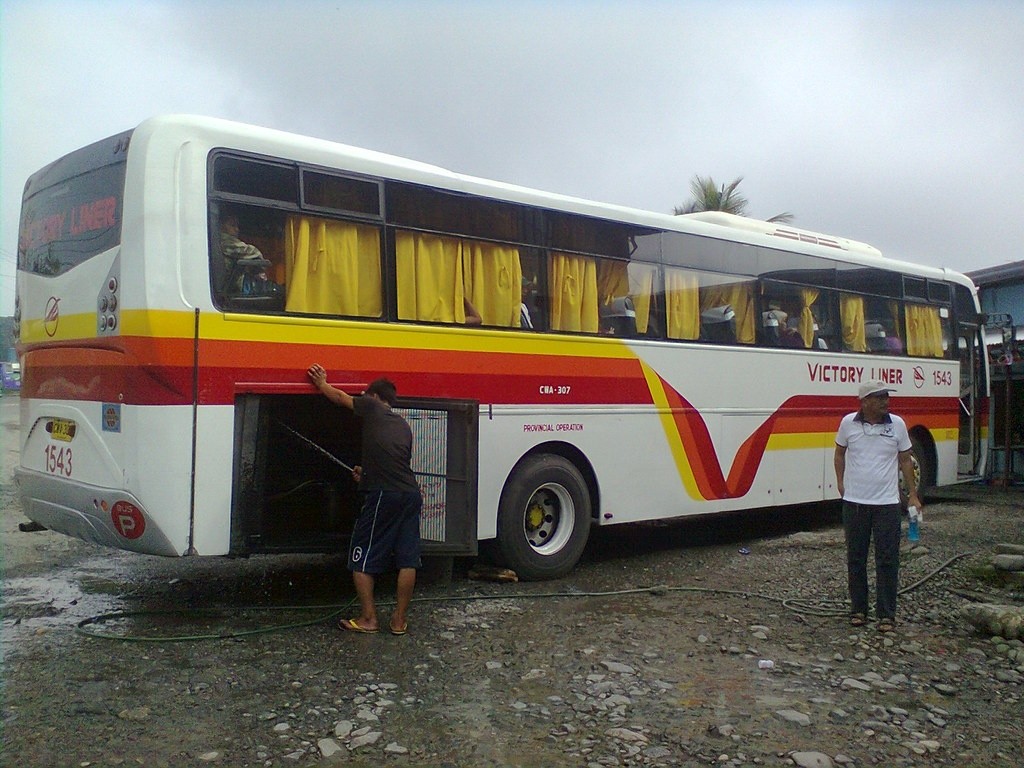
[{"xmin": 519, "ymin": 290, "xmax": 889, "ymax": 355}]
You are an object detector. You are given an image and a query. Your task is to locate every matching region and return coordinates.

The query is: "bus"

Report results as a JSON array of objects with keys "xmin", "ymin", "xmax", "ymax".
[{"xmin": 12, "ymin": 111, "xmax": 1018, "ymax": 578}]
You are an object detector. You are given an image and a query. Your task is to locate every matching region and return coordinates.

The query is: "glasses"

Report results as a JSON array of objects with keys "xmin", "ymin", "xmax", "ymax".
[{"xmin": 863, "ymin": 424, "xmax": 886, "ymax": 436}]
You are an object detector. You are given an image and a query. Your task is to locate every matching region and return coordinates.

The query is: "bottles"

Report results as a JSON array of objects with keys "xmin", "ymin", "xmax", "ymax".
[{"xmin": 905, "ymin": 512, "xmax": 920, "ymax": 543}]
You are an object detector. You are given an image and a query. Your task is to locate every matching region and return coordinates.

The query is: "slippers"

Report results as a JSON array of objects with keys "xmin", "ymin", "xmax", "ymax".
[
  {"xmin": 338, "ymin": 619, "xmax": 379, "ymax": 634},
  {"xmin": 389, "ymin": 620, "xmax": 407, "ymax": 634}
]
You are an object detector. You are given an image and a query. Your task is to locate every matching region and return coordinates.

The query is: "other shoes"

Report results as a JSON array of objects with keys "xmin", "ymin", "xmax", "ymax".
[
  {"xmin": 876, "ymin": 617, "xmax": 895, "ymax": 632},
  {"xmin": 851, "ymin": 612, "xmax": 870, "ymax": 627}
]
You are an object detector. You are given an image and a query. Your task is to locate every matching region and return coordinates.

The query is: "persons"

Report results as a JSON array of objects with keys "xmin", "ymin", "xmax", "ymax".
[
  {"xmin": 773, "ymin": 309, "xmax": 805, "ymax": 348},
  {"xmin": 812, "ymin": 313, "xmax": 828, "ymax": 350},
  {"xmin": 220, "ymin": 209, "xmax": 286, "ymax": 304},
  {"xmin": 308, "ymin": 363, "xmax": 423, "ymax": 635},
  {"xmin": 521, "ymin": 271, "xmax": 538, "ymax": 329},
  {"xmin": 834, "ymin": 380, "xmax": 921, "ymax": 632},
  {"xmin": 464, "ymin": 296, "xmax": 483, "ymax": 324}
]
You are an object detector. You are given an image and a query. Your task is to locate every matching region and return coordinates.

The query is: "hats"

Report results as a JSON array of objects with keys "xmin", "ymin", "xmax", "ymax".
[
  {"xmin": 859, "ymin": 380, "xmax": 897, "ymax": 400},
  {"xmin": 522, "ymin": 276, "xmax": 532, "ymax": 287}
]
